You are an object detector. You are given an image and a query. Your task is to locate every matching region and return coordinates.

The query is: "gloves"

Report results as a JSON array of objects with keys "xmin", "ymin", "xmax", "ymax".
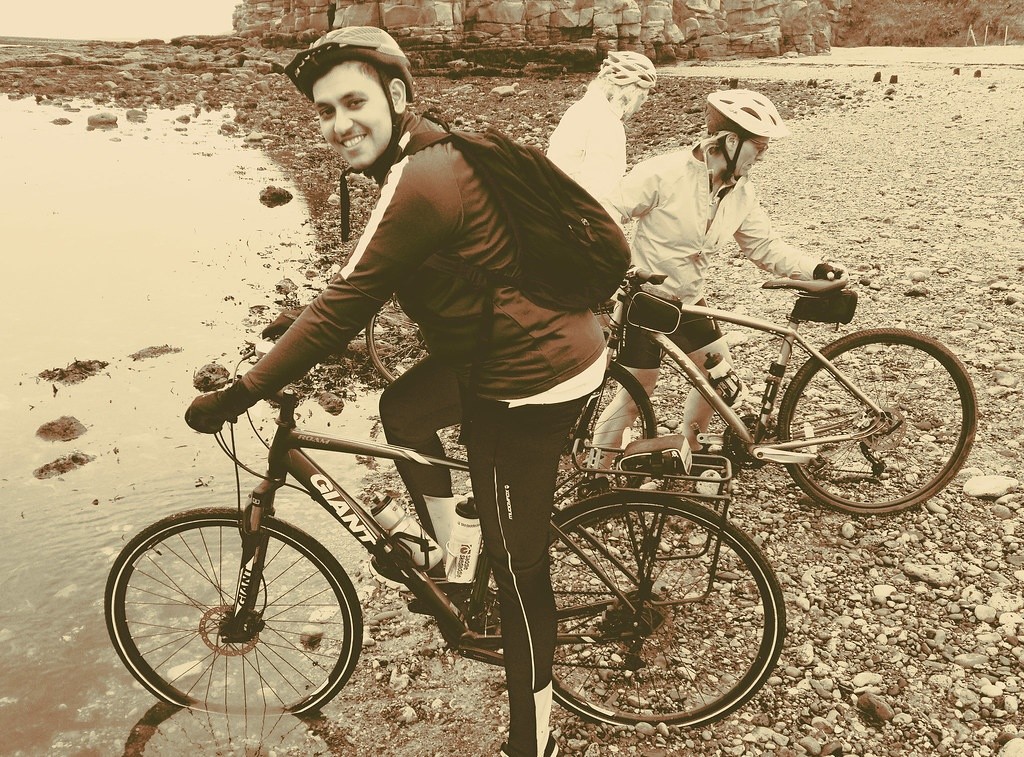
[{"xmin": 185, "ymin": 392, "xmax": 244, "ymax": 434}]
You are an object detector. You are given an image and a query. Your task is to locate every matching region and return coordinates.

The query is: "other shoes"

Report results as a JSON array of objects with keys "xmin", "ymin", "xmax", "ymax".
[
  {"xmin": 368, "ymin": 558, "xmax": 453, "ymax": 593},
  {"xmin": 498, "ymin": 735, "xmax": 560, "ymax": 757}
]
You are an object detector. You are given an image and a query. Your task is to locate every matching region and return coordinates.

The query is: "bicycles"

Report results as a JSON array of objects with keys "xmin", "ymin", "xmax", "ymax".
[
  {"xmin": 553, "ymin": 264, "xmax": 979, "ymax": 517},
  {"xmin": 105, "ymin": 336, "xmax": 787, "ymax": 731}
]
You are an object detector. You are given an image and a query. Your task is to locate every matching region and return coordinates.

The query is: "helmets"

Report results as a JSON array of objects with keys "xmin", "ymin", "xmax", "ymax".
[
  {"xmin": 598, "ymin": 51, "xmax": 657, "ymax": 88},
  {"xmin": 284, "ymin": 27, "xmax": 415, "ymax": 102},
  {"xmin": 706, "ymin": 89, "xmax": 790, "ymax": 138}
]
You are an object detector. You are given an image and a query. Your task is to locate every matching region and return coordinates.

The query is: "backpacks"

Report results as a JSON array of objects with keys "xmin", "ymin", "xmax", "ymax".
[{"xmin": 395, "ymin": 108, "xmax": 631, "ymax": 363}]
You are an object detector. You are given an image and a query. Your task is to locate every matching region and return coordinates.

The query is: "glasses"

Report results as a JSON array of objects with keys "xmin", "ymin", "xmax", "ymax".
[{"xmin": 746, "ymin": 135, "xmax": 768, "ymax": 155}]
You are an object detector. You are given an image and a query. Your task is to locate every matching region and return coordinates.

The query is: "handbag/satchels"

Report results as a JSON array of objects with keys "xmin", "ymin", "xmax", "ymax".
[{"xmin": 623, "ymin": 289, "xmax": 682, "ymax": 335}]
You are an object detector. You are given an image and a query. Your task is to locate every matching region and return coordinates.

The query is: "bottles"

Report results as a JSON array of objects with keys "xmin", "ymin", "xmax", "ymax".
[
  {"xmin": 445, "ymin": 497, "xmax": 481, "ymax": 583},
  {"xmin": 370, "ymin": 492, "xmax": 444, "ymax": 572},
  {"xmin": 703, "ymin": 352, "xmax": 750, "ymax": 408}
]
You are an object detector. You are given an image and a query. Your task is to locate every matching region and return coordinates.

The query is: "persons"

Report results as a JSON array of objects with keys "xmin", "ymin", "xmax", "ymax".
[
  {"xmin": 578, "ymin": 49, "xmax": 847, "ymax": 496},
  {"xmin": 545, "ymin": 50, "xmax": 657, "ymax": 328},
  {"xmin": 184, "ymin": 25, "xmax": 605, "ymax": 757}
]
